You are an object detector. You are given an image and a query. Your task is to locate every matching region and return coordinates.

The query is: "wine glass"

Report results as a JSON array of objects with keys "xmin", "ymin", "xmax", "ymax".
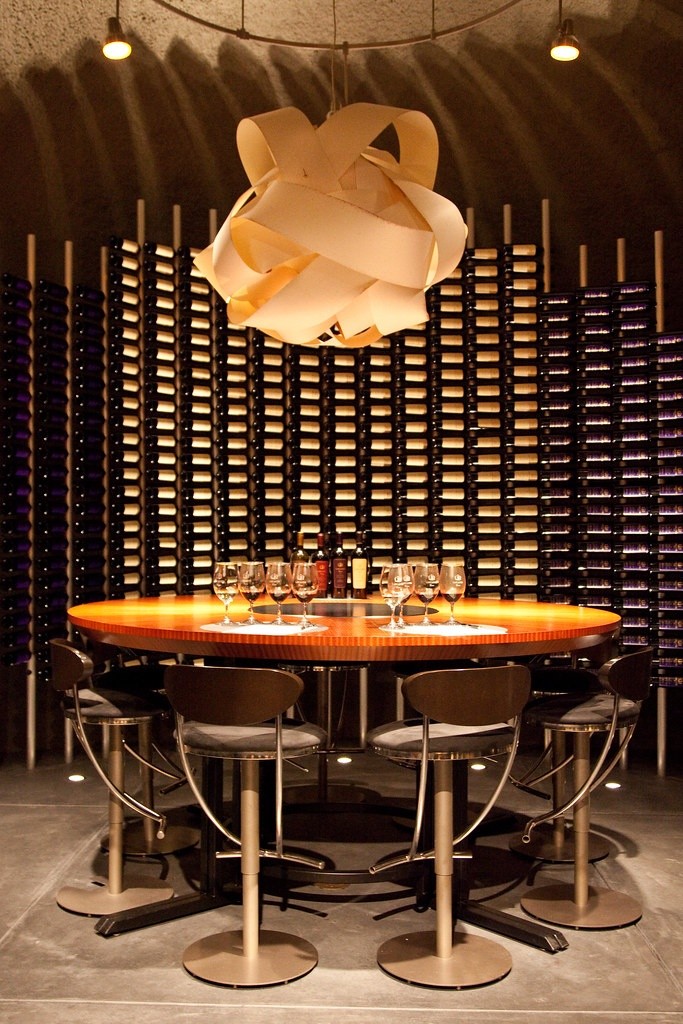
[
  {"xmin": 292, "ymin": 563, "xmax": 320, "ymax": 629},
  {"xmin": 213, "ymin": 561, "xmax": 239, "ymax": 626},
  {"xmin": 388, "ymin": 563, "xmax": 415, "ymax": 627},
  {"xmin": 379, "ymin": 564, "xmax": 406, "ymax": 631},
  {"xmin": 414, "ymin": 563, "xmax": 441, "ymax": 627},
  {"xmin": 266, "ymin": 563, "xmax": 292, "ymax": 627},
  {"xmin": 239, "ymin": 561, "xmax": 266, "ymax": 626},
  {"xmin": 439, "ymin": 564, "xmax": 467, "ymax": 626}
]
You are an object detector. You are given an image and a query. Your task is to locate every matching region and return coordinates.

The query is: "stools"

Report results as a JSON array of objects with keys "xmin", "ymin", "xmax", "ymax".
[
  {"xmin": 366, "ymin": 664, "xmax": 531, "ymax": 992},
  {"xmin": 47, "ymin": 637, "xmax": 174, "ymax": 919},
  {"xmin": 520, "ymin": 645, "xmax": 658, "ymax": 930},
  {"xmin": 155, "ymin": 660, "xmax": 327, "ymax": 987},
  {"xmin": 510, "ymin": 636, "xmax": 656, "ymax": 863},
  {"xmin": 77, "ymin": 640, "xmax": 202, "ymax": 858}
]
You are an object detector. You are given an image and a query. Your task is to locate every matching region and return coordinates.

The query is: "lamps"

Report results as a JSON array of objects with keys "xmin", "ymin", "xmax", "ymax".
[
  {"xmin": 104, "ymin": 2, "xmax": 132, "ymax": 61},
  {"xmin": 548, "ymin": 1, "xmax": 580, "ymax": 60}
]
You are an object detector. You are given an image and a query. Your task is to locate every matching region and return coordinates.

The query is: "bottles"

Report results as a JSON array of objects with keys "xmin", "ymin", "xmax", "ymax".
[
  {"xmin": 289, "ymin": 531, "xmax": 309, "ymax": 574},
  {"xmin": 329, "ymin": 532, "xmax": 348, "ymax": 599},
  {"xmin": 310, "ymin": 533, "xmax": 330, "ymax": 598},
  {"xmin": 350, "ymin": 530, "xmax": 370, "ymax": 599}
]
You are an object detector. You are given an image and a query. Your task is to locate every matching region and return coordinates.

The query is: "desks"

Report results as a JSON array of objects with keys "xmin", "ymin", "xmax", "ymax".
[{"xmin": 67, "ymin": 596, "xmax": 621, "ymax": 954}]
